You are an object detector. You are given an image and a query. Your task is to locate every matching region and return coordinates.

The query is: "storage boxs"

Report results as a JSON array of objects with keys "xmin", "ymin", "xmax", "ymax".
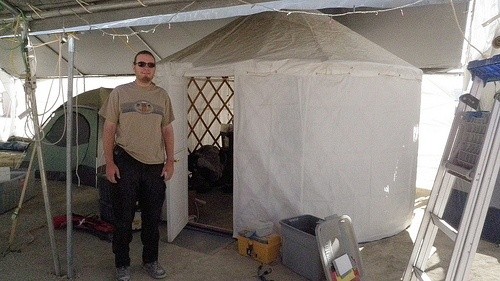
[
  {"xmin": 235, "ymin": 231, "xmax": 281, "ymax": 264},
  {"xmin": 278, "ymin": 213, "xmax": 362, "ymax": 281},
  {"xmin": 0, "ymin": 169, "xmax": 37, "ymax": 215}
]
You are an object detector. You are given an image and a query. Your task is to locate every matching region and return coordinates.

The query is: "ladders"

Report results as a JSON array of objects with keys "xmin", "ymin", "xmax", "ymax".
[{"xmin": 401, "ymin": 54, "xmax": 500, "ymax": 281}]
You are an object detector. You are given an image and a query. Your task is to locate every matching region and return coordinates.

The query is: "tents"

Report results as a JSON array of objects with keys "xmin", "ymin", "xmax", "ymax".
[
  {"xmin": 154, "ymin": 8, "xmax": 423, "ymax": 244},
  {"xmin": 35, "ymin": 87, "xmax": 114, "ymax": 188}
]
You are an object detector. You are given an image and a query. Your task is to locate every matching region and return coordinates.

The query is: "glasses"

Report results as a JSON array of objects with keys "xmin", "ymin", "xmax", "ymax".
[{"xmin": 134, "ymin": 61, "xmax": 155, "ymax": 68}]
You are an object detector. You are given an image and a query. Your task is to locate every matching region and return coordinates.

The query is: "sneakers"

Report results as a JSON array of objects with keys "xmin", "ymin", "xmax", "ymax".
[
  {"xmin": 112, "ymin": 265, "xmax": 132, "ymax": 281},
  {"xmin": 140, "ymin": 261, "xmax": 168, "ymax": 279}
]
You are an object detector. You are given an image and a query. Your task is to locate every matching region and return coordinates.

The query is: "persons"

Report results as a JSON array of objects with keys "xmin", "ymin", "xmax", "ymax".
[{"xmin": 98, "ymin": 50, "xmax": 175, "ymax": 281}]
